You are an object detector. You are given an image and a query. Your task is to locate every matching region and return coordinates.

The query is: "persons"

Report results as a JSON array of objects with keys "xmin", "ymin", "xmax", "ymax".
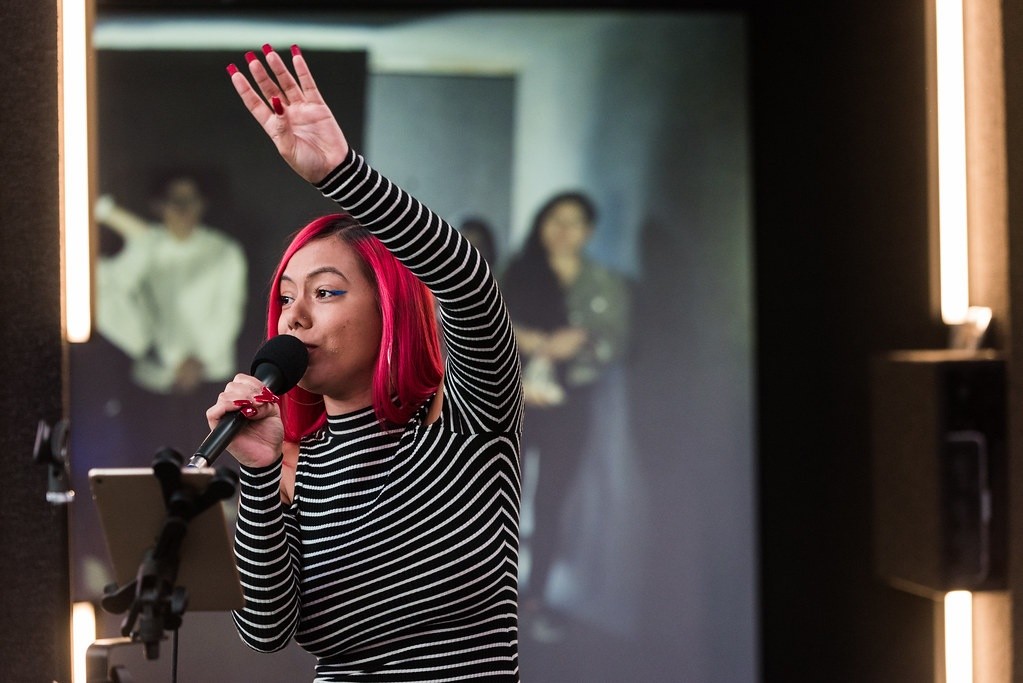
[
  {"xmin": 95, "ymin": 168, "xmax": 246, "ymax": 467},
  {"xmin": 205, "ymin": 44, "xmax": 525, "ymax": 683},
  {"xmin": 456, "ymin": 192, "xmax": 629, "ymax": 612}
]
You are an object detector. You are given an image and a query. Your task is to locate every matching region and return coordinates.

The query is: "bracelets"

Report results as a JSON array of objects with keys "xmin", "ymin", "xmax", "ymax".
[{"xmin": 94, "ymin": 193, "xmax": 114, "ymax": 222}]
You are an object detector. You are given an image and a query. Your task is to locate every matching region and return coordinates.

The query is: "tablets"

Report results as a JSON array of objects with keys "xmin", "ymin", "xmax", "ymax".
[{"xmin": 86, "ymin": 466, "xmax": 245, "ymax": 613}]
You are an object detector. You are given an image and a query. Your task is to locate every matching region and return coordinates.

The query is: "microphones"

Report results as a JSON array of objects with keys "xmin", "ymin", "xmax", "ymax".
[{"xmin": 192, "ymin": 334, "xmax": 309, "ymax": 470}]
